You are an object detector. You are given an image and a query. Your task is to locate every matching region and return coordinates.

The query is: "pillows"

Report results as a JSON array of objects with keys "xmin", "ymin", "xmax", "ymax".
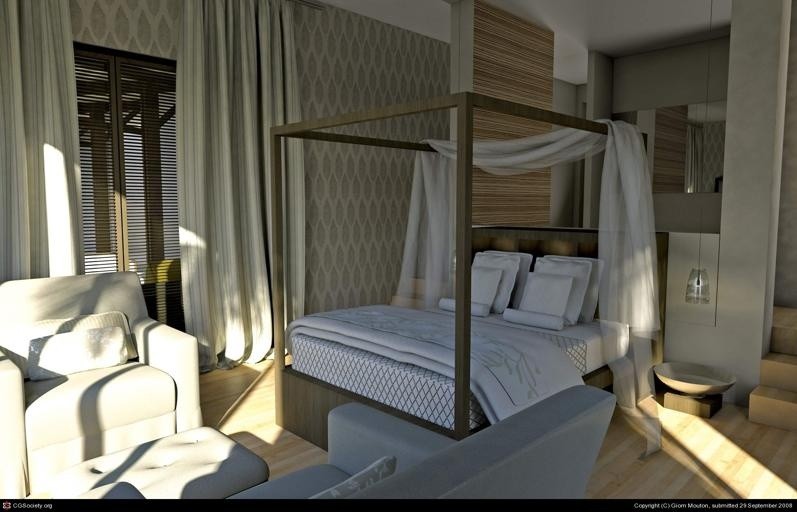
[
  {"xmin": 473, "ymin": 252, "xmax": 520, "ymax": 314},
  {"xmin": 519, "ymin": 271, "xmax": 573, "ymax": 315},
  {"xmin": 544, "ymin": 255, "xmax": 604, "ymax": 324},
  {"xmin": 471, "ymin": 266, "xmax": 502, "ymax": 307},
  {"xmin": 482, "ymin": 250, "xmax": 534, "ymax": 311},
  {"xmin": 439, "ymin": 297, "xmax": 489, "ymax": 317},
  {"xmin": 8, "ymin": 311, "xmax": 138, "ymax": 381},
  {"xmin": 534, "ymin": 257, "xmax": 593, "ymax": 324},
  {"xmin": 311, "ymin": 455, "xmax": 398, "ymax": 499},
  {"xmin": 503, "ymin": 307, "xmax": 564, "ymax": 331}
]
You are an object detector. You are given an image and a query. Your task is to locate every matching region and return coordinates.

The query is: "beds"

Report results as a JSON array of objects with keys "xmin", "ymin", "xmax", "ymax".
[{"xmin": 270, "ymin": 91, "xmax": 669, "ymax": 455}]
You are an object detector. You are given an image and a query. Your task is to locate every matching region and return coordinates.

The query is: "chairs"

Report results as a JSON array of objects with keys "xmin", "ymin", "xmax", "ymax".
[
  {"xmin": 0, "ymin": 271, "xmax": 203, "ymax": 501},
  {"xmin": 223, "ymin": 385, "xmax": 617, "ymax": 499}
]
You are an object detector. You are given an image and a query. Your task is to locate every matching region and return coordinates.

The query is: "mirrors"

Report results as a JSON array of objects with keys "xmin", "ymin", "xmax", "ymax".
[{"xmin": 589, "ymin": 98, "xmax": 728, "ymax": 234}]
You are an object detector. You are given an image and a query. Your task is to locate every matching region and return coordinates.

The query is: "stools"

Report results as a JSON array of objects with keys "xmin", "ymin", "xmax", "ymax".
[{"xmin": 33, "ymin": 425, "xmax": 269, "ymax": 500}]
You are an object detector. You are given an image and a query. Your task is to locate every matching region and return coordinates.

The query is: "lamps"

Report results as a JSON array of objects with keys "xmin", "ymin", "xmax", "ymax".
[{"xmin": 686, "ymin": 0, "xmax": 719, "ymax": 306}]
